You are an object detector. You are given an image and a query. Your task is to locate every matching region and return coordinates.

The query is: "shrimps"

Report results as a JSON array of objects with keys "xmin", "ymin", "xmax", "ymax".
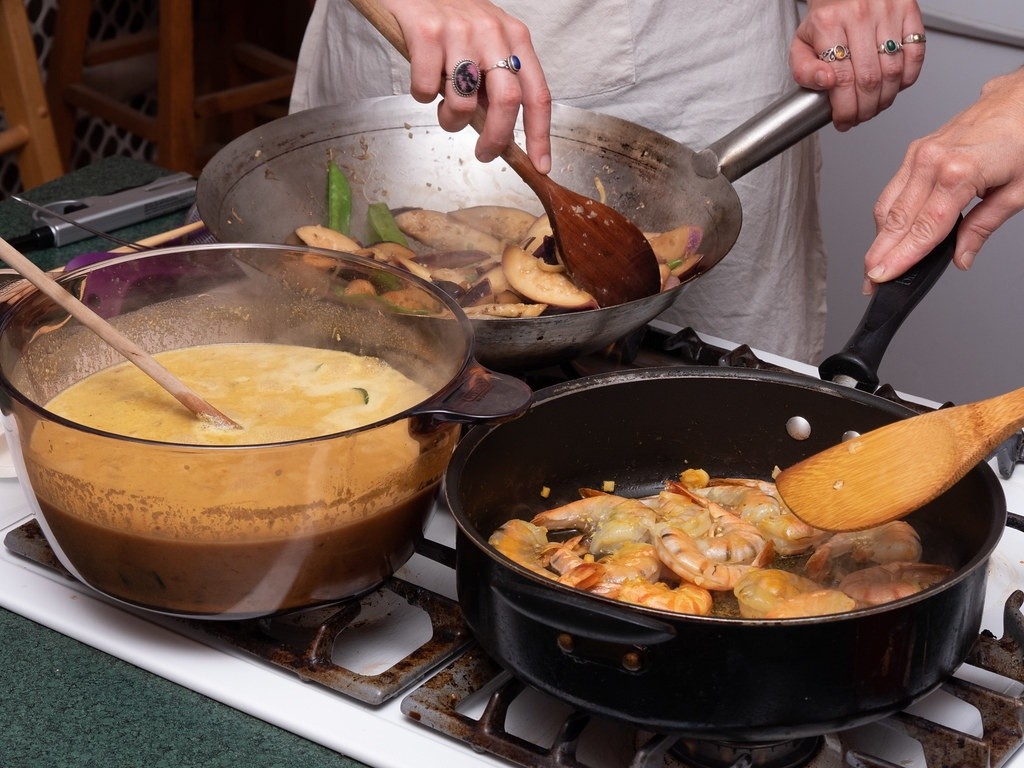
[{"xmin": 488, "ymin": 478, "xmax": 956, "ymax": 619}]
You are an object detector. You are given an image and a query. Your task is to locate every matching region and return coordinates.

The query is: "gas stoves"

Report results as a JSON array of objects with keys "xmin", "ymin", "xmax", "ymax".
[{"xmin": 0, "ymin": 321, "xmax": 1024, "ymax": 767}]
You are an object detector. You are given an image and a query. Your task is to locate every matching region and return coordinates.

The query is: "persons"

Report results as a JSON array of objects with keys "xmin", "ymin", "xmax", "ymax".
[
  {"xmin": 863, "ymin": 63, "xmax": 1024, "ymax": 297},
  {"xmin": 288, "ymin": 0, "xmax": 925, "ymax": 365}
]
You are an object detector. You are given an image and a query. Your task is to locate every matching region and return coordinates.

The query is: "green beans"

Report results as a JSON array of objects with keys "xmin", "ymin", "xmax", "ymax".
[{"xmin": 327, "ymin": 161, "xmax": 431, "ymax": 315}]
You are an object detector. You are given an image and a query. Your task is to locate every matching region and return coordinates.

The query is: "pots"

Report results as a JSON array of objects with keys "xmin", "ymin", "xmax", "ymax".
[
  {"xmin": 436, "ymin": 211, "xmax": 1007, "ymax": 743},
  {"xmin": 196, "ymin": 83, "xmax": 832, "ymax": 360},
  {"xmin": 0, "ymin": 241, "xmax": 534, "ymax": 623}
]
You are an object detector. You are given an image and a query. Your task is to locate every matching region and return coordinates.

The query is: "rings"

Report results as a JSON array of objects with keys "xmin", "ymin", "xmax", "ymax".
[
  {"xmin": 443, "ymin": 59, "xmax": 481, "ymax": 96},
  {"xmin": 817, "ymin": 44, "xmax": 851, "ymax": 62},
  {"xmin": 875, "ymin": 39, "xmax": 904, "ymax": 55},
  {"xmin": 901, "ymin": 33, "xmax": 927, "ymax": 45},
  {"xmin": 481, "ymin": 54, "xmax": 522, "ymax": 74}
]
